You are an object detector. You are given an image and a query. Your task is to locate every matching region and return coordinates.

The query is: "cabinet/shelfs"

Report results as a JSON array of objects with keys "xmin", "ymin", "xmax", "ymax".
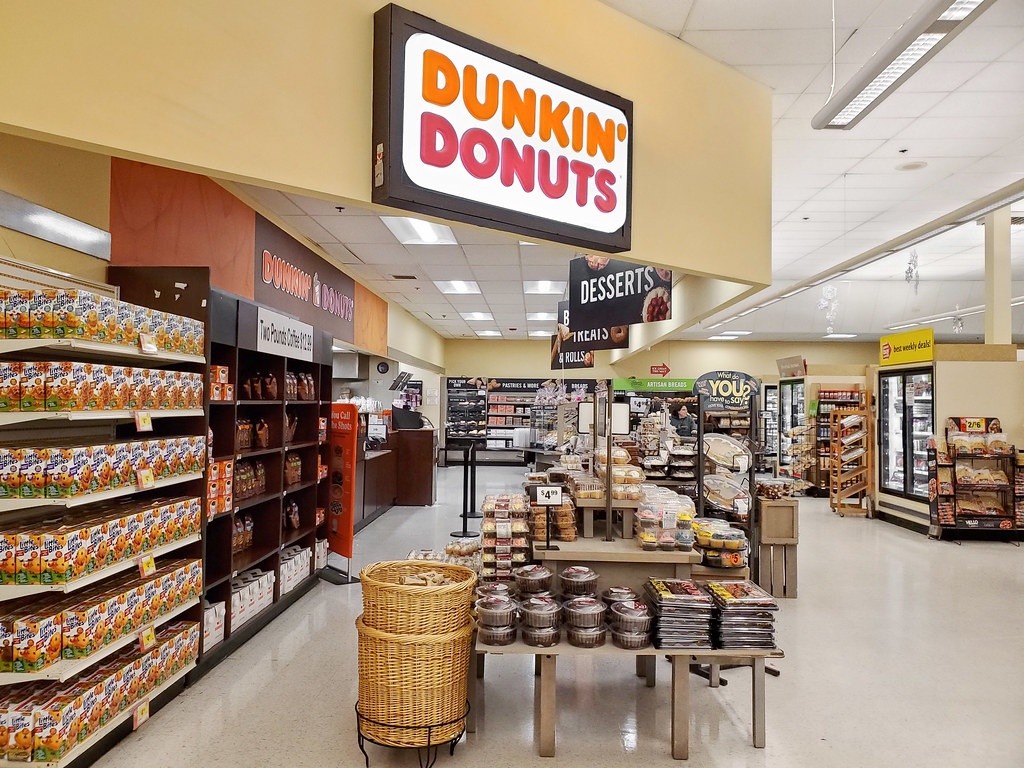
[
  {"xmin": 115, "ymin": 286, "xmax": 334, "ymax": 655},
  {"xmin": 0, "ymin": 256, "xmax": 207, "ymax": 768},
  {"xmin": 924, "ymin": 444, "xmax": 1016, "ymax": 528},
  {"xmin": 810, "ymin": 382, "xmax": 866, "ymax": 495},
  {"xmin": 485, "ymin": 400, "xmax": 533, "ymax": 450}
]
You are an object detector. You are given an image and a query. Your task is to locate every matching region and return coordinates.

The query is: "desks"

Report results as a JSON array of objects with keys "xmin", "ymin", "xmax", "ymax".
[
  {"xmin": 474, "ymin": 620, "xmax": 785, "ymax": 747},
  {"xmin": 532, "ymin": 521, "xmax": 702, "ymax": 759}
]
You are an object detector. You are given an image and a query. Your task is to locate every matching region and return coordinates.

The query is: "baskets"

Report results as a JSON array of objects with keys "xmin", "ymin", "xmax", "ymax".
[
  {"xmin": 355, "ymin": 613, "xmax": 475, "ymax": 748},
  {"xmin": 359, "ymin": 560, "xmax": 477, "ymax": 634}
]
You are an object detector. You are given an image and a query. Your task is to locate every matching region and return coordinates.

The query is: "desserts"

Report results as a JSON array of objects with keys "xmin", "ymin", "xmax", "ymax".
[{"xmin": 478, "ymin": 447, "xmax": 747, "ymax": 582}]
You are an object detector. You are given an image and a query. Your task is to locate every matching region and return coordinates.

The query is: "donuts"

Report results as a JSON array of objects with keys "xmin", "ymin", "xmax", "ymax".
[{"xmin": 610, "ymin": 325, "xmax": 627, "ymax": 343}]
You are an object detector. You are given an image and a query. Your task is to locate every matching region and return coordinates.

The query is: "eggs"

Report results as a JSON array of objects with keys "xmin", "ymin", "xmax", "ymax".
[{"xmin": 446, "ymin": 541, "xmax": 479, "ymax": 556}]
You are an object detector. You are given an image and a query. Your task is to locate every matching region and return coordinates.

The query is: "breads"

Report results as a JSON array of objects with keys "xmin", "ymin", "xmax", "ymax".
[{"xmin": 585, "ymin": 254, "xmax": 610, "ymax": 271}]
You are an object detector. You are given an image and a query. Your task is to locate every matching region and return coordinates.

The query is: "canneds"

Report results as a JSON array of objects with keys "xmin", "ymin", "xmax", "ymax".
[{"xmin": 819, "ymin": 391, "xmax": 860, "ymax": 488}]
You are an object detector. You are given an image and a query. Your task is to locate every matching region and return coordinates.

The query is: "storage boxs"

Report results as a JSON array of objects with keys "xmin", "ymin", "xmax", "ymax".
[
  {"xmin": 316, "ymin": 507, "xmax": 325, "ymax": 525},
  {"xmin": 279, "ymin": 545, "xmax": 311, "ymax": 598},
  {"xmin": 318, "ymin": 417, "xmax": 327, "ymax": 441},
  {"xmin": 206, "ymin": 459, "xmax": 234, "ymax": 516},
  {"xmin": 490, "ymin": 405, "xmax": 514, "ymax": 415},
  {"xmin": 315, "ymin": 539, "xmax": 329, "ymax": 572},
  {"xmin": 516, "ymin": 408, "xmax": 523, "ymax": 414},
  {"xmin": 481, "ymin": 447, "xmax": 698, "ymax": 580},
  {"xmin": 231, "ymin": 568, "xmax": 274, "ymax": 633},
  {"xmin": 488, "ymin": 416, "xmax": 522, "ymax": 425},
  {"xmin": 525, "ymin": 407, "xmax": 530, "ymax": 414},
  {"xmin": 209, "ymin": 364, "xmax": 234, "ymax": 400},
  {"xmin": 523, "ymin": 419, "xmax": 530, "ymax": 427},
  {"xmin": 490, "ymin": 395, "xmax": 506, "ymax": 402},
  {"xmin": 0, "ymin": 435, "xmax": 206, "ymax": 762},
  {"xmin": 756, "ymin": 496, "xmax": 800, "ymax": 544},
  {"xmin": 0, "ymin": 289, "xmax": 203, "ymax": 357},
  {"xmin": 203, "ymin": 601, "xmax": 226, "ymax": 653},
  {"xmin": 317, "ymin": 455, "xmax": 327, "ymax": 479},
  {"xmin": 0, "ymin": 361, "xmax": 204, "ymax": 411}
]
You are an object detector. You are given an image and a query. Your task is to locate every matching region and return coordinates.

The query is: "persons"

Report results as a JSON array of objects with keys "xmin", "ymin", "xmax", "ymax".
[{"xmin": 671, "ymin": 404, "xmax": 697, "ymax": 436}]
